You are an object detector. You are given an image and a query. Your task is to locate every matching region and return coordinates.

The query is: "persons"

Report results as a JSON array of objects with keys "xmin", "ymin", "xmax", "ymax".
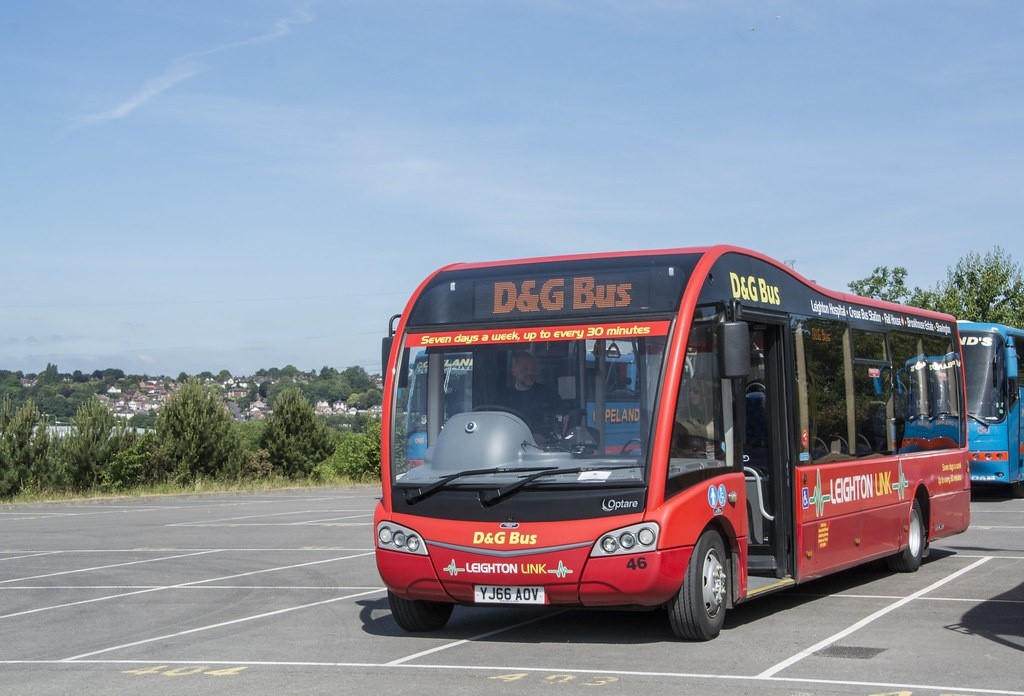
[
  {"xmin": 488, "ymin": 352, "xmax": 562, "ymax": 444},
  {"xmin": 744, "ymin": 379, "xmax": 765, "ymax": 394},
  {"xmin": 675, "ymin": 376, "xmax": 715, "ymax": 439}
]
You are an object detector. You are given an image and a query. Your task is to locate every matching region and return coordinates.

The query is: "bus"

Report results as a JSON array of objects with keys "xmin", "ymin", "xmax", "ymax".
[
  {"xmin": 375, "ymin": 244, "xmax": 971, "ymax": 642},
  {"xmin": 895, "ymin": 352, "xmax": 961, "ymax": 455},
  {"xmin": 956, "ymin": 320, "xmax": 1024, "ymax": 498}
]
